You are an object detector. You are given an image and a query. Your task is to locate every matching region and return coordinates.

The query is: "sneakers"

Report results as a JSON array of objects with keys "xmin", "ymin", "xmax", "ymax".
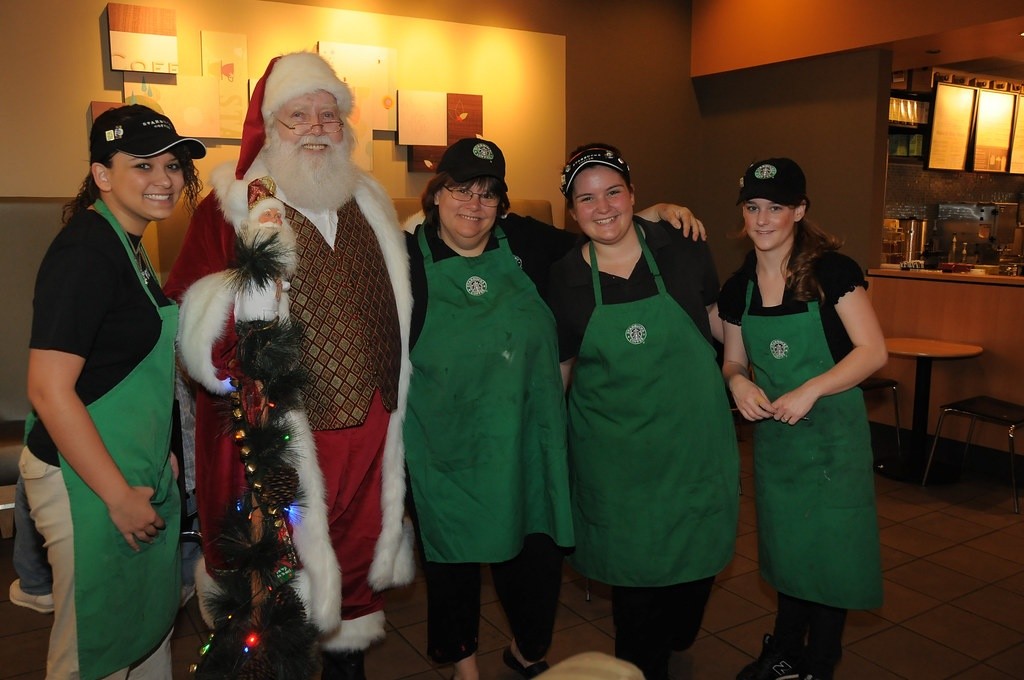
[
  {"xmin": 179, "ymin": 585, "xmax": 196, "ymax": 608},
  {"xmin": 735, "ymin": 633, "xmax": 816, "ymax": 680},
  {"xmin": 10, "ymin": 579, "xmax": 56, "ymax": 613}
]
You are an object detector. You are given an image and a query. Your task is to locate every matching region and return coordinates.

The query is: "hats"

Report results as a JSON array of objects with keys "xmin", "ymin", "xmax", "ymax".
[
  {"xmin": 436, "ymin": 137, "xmax": 508, "ymax": 192},
  {"xmin": 735, "ymin": 158, "xmax": 805, "ymax": 207},
  {"xmin": 90, "ymin": 104, "xmax": 207, "ymax": 164},
  {"xmin": 228, "ymin": 51, "xmax": 353, "ymax": 210}
]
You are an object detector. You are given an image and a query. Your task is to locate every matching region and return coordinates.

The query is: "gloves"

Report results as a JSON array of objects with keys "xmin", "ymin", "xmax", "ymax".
[{"xmin": 233, "ymin": 276, "xmax": 291, "ymax": 323}]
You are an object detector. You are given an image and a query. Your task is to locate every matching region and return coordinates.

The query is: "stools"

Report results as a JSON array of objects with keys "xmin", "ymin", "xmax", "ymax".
[
  {"xmin": 855, "ymin": 376, "xmax": 902, "ymax": 457},
  {"xmin": 921, "ymin": 395, "xmax": 1024, "ymax": 514}
]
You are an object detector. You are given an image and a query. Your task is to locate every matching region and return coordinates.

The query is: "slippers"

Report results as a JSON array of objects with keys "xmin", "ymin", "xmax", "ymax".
[{"xmin": 502, "ymin": 644, "xmax": 550, "ymax": 680}]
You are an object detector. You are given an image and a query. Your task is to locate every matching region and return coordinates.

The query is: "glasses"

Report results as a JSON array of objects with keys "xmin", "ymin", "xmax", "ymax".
[
  {"xmin": 272, "ymin": 112, "xmax": 344, "ymax": 137},
  {"xmin": 442, "ymin": 183, "xmax": 501, "ymax": 207}
]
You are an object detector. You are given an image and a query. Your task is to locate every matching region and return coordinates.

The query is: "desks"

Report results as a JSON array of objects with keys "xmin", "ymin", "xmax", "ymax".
[{"xmin": 882, "ymin": 338, "xmax": 983, "ymax": 475}]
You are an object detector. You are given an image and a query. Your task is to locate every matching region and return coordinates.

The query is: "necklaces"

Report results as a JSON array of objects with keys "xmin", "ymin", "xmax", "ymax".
[{"xmin": 123, "ymin": 229, "xmax": 151, "ymax": 284}]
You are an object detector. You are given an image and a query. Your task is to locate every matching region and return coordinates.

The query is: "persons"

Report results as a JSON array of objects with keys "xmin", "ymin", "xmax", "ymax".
[
  {"xmin": 9, "ymin": 103, "xmax": 207, "ymax": 680},
  {"xmin": 164, "ymin": 50, "xmax": 415, "ymax": 680},
  {"xmin": 396, "ymin": 139, "xmax": 708, "ymax": 680},
  {"xmin": 718, "ymin": 158, "xmax": 889, "ymax": 680},
  {"xmin": 547, "ymin": 143, "xmax": 742, "ymax": 680}
]
